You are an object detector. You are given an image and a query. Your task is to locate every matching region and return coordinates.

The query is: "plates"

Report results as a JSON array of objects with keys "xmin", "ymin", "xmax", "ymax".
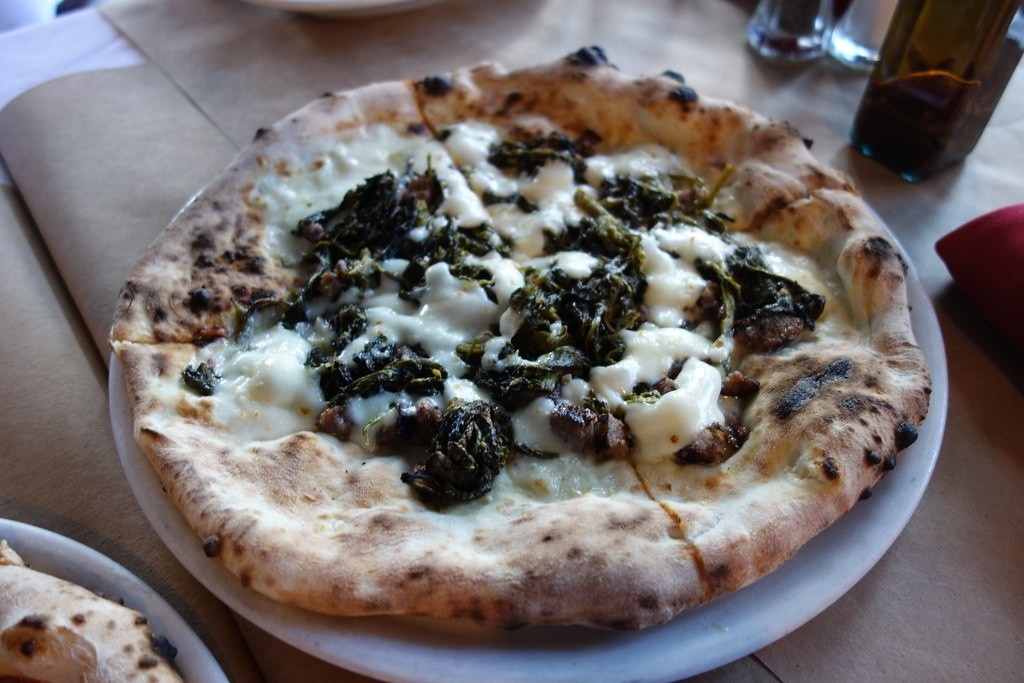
[
  {"xmin": 109, "ymin": 185, "xmax": 948, "ymax": 683},
  {"xmin": 0, "ymin": 518, "xmax": 230, "ymax": 683}
]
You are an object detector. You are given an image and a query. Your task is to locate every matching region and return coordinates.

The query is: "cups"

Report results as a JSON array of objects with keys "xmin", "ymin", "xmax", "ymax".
[{"xmin": 826, "ymin": 0, "xmax": 1024, "ymax": 187}]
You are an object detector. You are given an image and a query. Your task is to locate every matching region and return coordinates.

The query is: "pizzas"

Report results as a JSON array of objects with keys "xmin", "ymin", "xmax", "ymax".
[
  {"xmin": 0, "ymin": 538, "xmax": 189, "ymax": 683},
  {"xmin": 105, "ymin": 45, "xmax": 932, "ymax": 629}
]
